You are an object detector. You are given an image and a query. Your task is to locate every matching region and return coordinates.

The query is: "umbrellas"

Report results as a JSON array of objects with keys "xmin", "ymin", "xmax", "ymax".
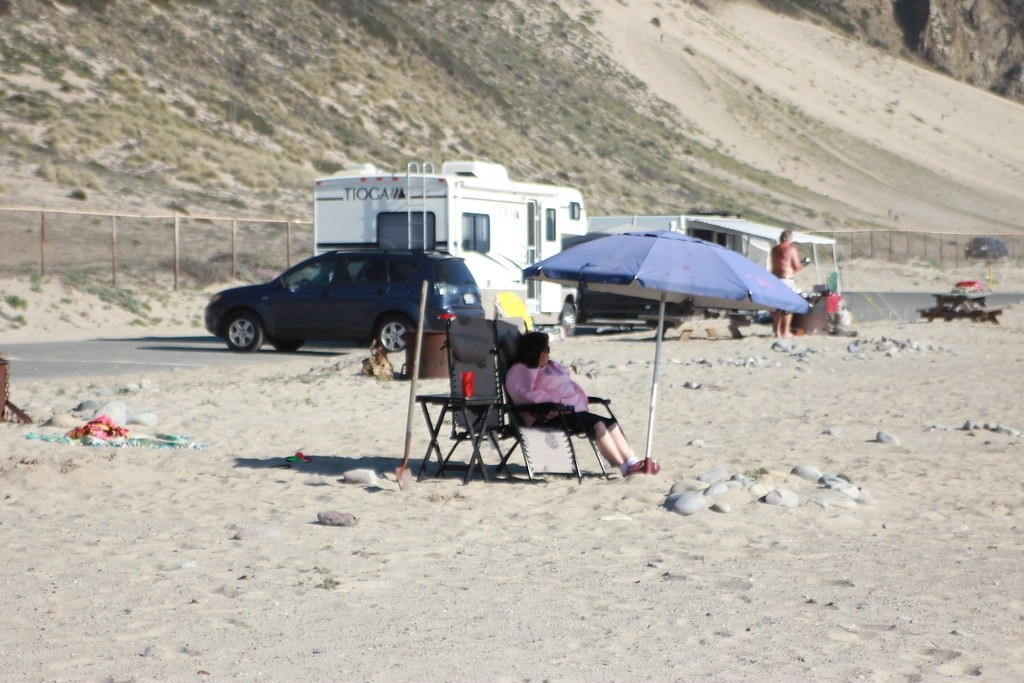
[{"xmin": 523, "ymin": 221, "xmax": 810, "ymax": 477}]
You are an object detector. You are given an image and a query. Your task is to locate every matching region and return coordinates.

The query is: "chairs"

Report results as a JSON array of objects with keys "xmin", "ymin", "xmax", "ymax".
[
  {"xmin": 494, "ymin": 319, "xmax": 635, "ymax": 481},
  {"xmin": 435, "ymin": 317, "xmax": 583, "ymax": 486}
]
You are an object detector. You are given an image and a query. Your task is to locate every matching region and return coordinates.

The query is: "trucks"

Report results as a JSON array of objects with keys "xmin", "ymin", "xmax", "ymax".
[{"xmin": 313, "ymin": 159, "xmax": 588, "ymax": 337}]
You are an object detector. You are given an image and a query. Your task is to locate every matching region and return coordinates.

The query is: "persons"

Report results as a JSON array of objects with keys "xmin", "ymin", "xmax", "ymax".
[
  {"xmin": 770, "ymin": 230, "xmax": 811, "ymax": 338},
  {"xmin": 505, "ymin": 332, "xmax": 660, "ymax": 479}
]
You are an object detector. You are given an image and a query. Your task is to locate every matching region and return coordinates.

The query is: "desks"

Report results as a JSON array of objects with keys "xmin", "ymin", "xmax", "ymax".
[
  {"xmin": 414, "ymin": 393, "xmax": 501, "ymax": 484},
  {"xmin": 927, "ymin": 292, "xmax": 1000, "ymax": 324}
]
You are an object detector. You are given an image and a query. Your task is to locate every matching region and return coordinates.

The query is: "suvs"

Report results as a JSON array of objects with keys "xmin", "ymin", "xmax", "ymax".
[{"xmin": 205, "ymin": 245, "xmax": 526, "ymax": 354}]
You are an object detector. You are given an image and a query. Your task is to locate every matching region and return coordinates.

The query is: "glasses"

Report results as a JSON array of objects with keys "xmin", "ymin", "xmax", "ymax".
[{"xmin": 541, "ymin": 346, "xmax": 550, "ymax": 353}]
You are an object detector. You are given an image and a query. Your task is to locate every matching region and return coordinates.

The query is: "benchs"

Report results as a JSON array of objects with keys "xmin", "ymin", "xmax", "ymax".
[
  {"xmin": 917, "ymin": 305, "xmax": 950, "ymax": 313},
  {"xmin": 974, "ymin": 306, "xmax": 1003, "ymax": 315}
]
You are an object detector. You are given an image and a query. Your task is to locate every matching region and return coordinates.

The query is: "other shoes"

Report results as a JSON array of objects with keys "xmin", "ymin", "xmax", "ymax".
[
  {"xmin": 623, "ymin": 458, "xmax": 652, "ymax": 477},
  {"xmin": 650, "ymin": 461, "xmax": 661, "ymax": 475}
]
